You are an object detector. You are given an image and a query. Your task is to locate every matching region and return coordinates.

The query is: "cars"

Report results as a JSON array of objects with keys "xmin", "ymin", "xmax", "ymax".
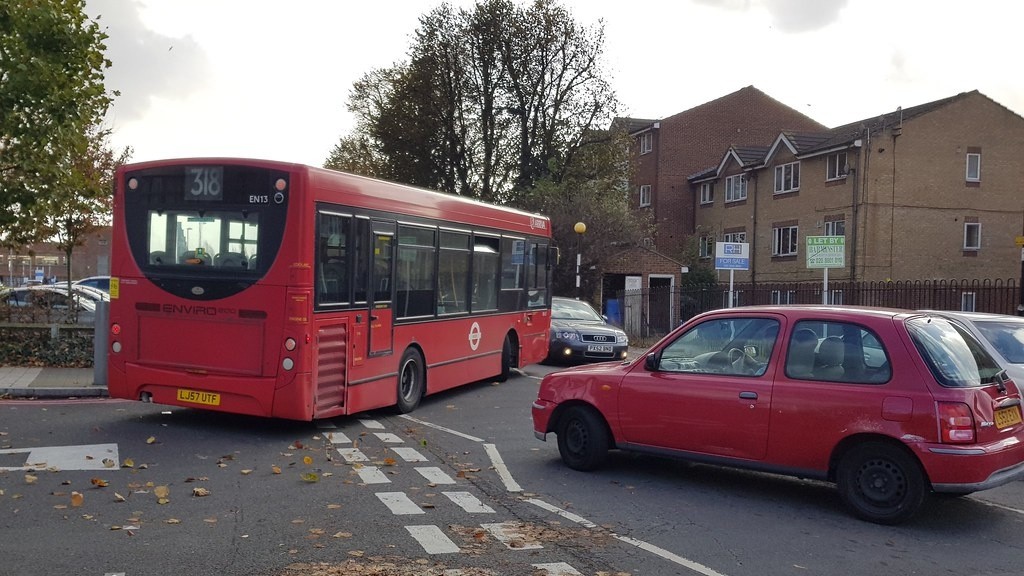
[
  {"xmin": 530, "ymin": 303, "xmax": 1024, "ymax": 526},
  {"xmin": 811, "ymin": 311, "xmax": 1024, "ymax": 415},
  {"xmin": 0, "ymin": 275, "xmax": 110, "ymax": 326},
  {"xmin": 530, "ymin": 295, "xmax": 629, "ymax": 368}
]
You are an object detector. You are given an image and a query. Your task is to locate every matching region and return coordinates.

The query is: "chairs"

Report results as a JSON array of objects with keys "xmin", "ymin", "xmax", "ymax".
[
  {"xmin": 148, "ymin": 250, "xmax": 257, "ymax": 270},
  {"xmin": 786, "ymin": 328, "xmax": 819, "ymax": 380},
  {"xmin": 813, "ymin": 335, "xmax": 845, "ymax": 381}
]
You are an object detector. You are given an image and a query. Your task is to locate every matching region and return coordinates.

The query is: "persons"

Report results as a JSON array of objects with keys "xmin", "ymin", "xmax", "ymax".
[{"xmin": 743, "ymin": 325, "xmax": 779, "ymax": 377}]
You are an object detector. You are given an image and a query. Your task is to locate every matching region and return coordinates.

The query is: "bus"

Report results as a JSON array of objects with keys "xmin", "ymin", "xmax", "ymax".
[{"xmin": 107, "ymin": 157, "xmax": 561, "ymax": 422}]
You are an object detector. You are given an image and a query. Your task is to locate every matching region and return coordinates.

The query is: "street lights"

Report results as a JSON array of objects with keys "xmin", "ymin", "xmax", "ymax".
[{"xmin": 574, "ymin": 222, "xmax": 586, "ymax": 299}]
